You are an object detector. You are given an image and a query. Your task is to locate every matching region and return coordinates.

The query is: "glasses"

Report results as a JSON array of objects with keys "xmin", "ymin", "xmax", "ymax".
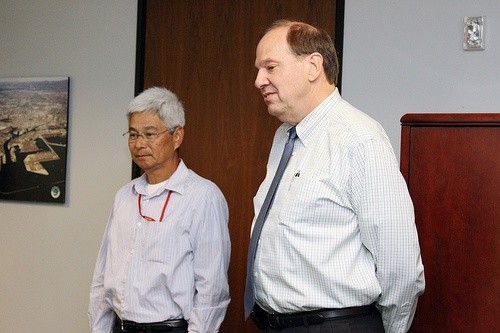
[{"xmin": 122, "ymin": 128, "xmax": 171, "ymax": 143}]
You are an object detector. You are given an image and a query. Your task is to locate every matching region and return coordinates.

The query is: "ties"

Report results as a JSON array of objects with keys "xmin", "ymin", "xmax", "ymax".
[{"xmin": 243, "ymin": 126, "xmax": 297, "ymax": 321}]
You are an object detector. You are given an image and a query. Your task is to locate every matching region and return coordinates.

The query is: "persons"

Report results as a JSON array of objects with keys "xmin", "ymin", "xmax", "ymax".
[
  {"xmin": 248, "ymin": 17, "xmax": 426, "ymax": 333},
  {"xmin": 89, "ymin": 87, "xmax": 232, "ymax": 333}
]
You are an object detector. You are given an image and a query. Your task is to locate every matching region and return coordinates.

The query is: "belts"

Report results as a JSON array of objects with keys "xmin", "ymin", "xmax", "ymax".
[
  {"xmin": 249, "ymin": 304, "xmax": 376, "ymax": 326},
  {"xmin": 112, "ymin": 318, "xmax": 187, "ymax": 333}
]
